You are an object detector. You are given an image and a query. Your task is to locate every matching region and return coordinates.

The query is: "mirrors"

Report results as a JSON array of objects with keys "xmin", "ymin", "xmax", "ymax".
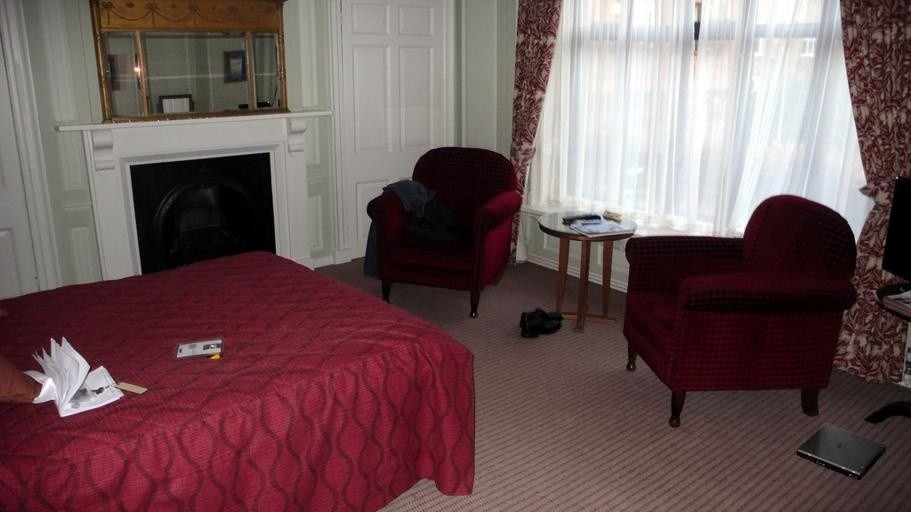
[{"xmin": 96, "ymin": 29, "xmax": 289, "ymax": 123}]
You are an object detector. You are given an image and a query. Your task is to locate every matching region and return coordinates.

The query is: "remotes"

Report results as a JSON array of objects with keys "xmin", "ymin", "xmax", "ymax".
[{"xmin": 562, "ymin": 214, "xmax": 601, "ymax": 224}]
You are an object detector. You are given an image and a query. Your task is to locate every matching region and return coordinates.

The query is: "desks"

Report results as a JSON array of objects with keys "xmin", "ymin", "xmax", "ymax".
[{"xmin": 539, "ymin": 210, "xmax": 637, "ymax": 334}]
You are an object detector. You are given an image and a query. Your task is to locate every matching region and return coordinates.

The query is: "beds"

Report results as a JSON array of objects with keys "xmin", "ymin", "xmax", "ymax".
[{"xmin": 0, "ymin": 251, "xmax": 468, "ymax": 512}]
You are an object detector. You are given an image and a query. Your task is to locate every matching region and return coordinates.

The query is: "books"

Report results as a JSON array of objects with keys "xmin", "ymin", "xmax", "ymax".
[
  {"xmin": 570, "ymin": 217, "xmax": 635, "ymax": 239},
  {"xmin": 883, "ymin": 290, "xmax": 911, "ymax": 317}
]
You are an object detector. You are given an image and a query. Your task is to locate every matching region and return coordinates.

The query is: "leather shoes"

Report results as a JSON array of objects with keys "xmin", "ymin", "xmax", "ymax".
[{"xmin": 519, "ymin": 307, "xmax": 563, "ymax": 340}]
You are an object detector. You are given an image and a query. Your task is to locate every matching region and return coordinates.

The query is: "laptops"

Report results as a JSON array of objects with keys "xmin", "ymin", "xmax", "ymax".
[{"xmin": 797, "ymin": 423, "xmax": 886, "ymax": 480}]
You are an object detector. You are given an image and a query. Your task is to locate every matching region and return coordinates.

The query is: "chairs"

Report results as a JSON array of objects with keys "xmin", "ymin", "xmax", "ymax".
[
  {"xmin": 363, "ymin": 146, "xmax": 523, "ymax": 319},
  {"xmin": 623, "ymin": 195, "xmax": 857, "ymax": 427}
]
